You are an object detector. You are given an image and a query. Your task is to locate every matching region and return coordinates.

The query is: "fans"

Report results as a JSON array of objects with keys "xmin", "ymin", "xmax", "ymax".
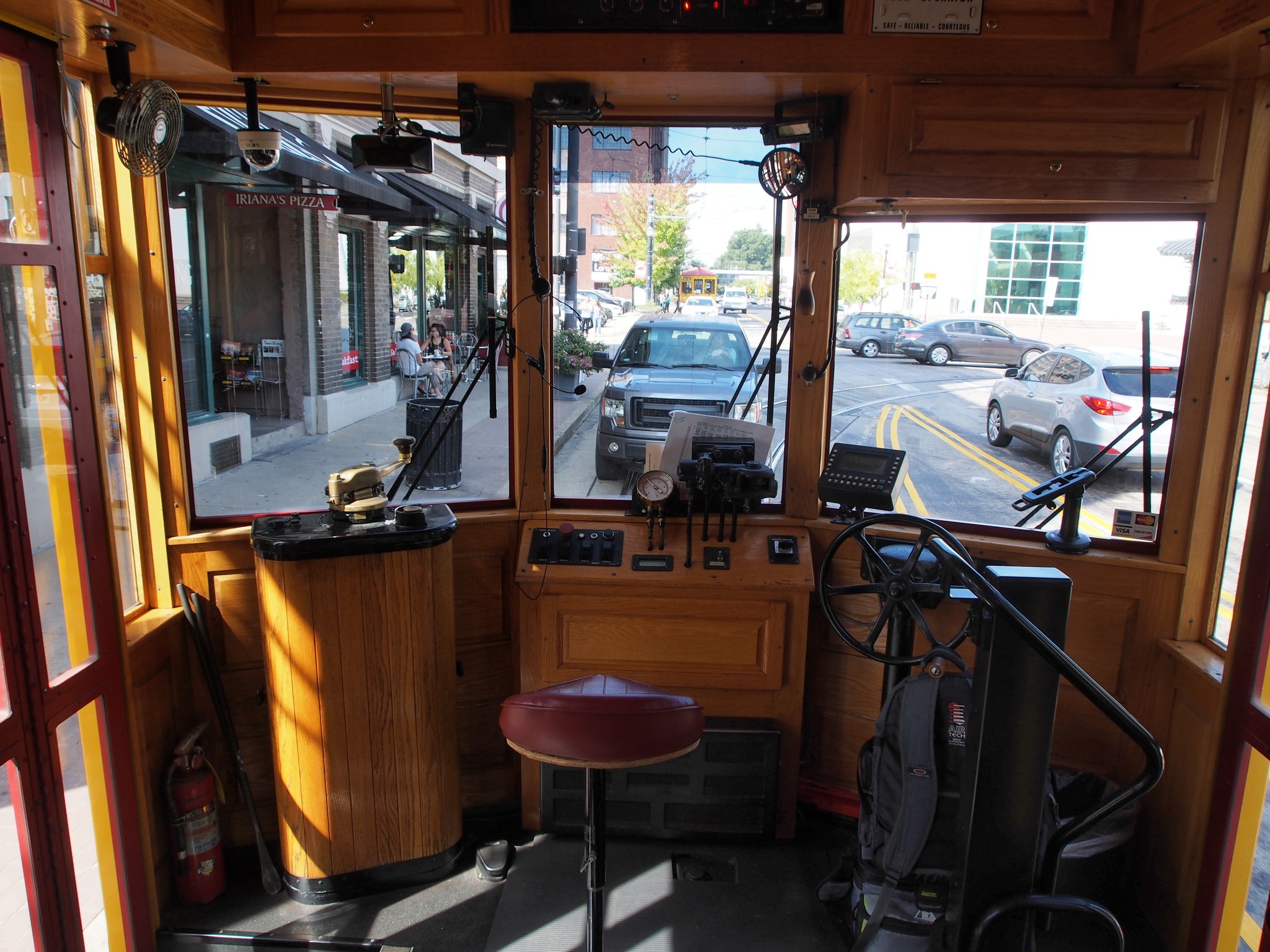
[{"xmin": 95, "ymin": 31, "xmax": 182, "ymax": 176}]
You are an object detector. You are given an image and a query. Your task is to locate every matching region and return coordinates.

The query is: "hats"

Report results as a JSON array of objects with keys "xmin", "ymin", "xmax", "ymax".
[{"xmin": 401, "ymin": 323, "xmax": 412, "ymax": 336}]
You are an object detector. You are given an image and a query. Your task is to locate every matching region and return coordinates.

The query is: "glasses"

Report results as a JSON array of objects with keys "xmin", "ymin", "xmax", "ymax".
[
  {"xmin": 410, "ymin": 328, "xmax": 414, "ymax": 331},
  {"xmin": 430, "ymin": 330, "xmax": 438, "ymax": 335}
]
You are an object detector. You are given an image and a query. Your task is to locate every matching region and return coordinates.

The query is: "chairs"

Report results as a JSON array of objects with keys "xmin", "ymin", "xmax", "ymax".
[
  {"xmin": 636, "ymin": 343, "xmax": 673, "ymax": 361},
  {"xmin": 396, "ymin": 348, "xmax": 429, "ymax": 401},
  {"xmin": 448, "ymin": 333, "xmax": 503, "ymax": 386}
]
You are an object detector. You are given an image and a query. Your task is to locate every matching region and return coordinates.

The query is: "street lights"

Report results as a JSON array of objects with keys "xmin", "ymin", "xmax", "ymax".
[{"xmin": 879, "ymin": 244, "xmax": 889, "ymax": 312}]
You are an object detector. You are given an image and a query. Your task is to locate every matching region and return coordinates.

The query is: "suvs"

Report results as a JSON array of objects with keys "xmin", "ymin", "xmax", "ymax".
[
  {"xmin": 986, "ymin": 346, "xmax": 1181, "ymax": 477},
  {"xmin": 592, "ymin": 313, "xmax": 782, "ymax": 482},
  {"xmin": 836, "ymin": 312, "xmax": 926, "ymax": 358},
  {"xmin": 721, "ymin": 287, "xmax": 749, "ymax": 314}
]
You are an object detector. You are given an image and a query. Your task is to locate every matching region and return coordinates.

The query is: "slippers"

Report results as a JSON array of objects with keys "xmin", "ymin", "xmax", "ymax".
[
  {"xmin": 440, "ymin": 381, "xmax": 444, "ymax": 384},
  {"xmin": 428, "ymin": 390, "xmax": 434, "ymax": 394},
  {"xmin": 417, "ymin": 386, "xmax": 426, "ymax": 393}
]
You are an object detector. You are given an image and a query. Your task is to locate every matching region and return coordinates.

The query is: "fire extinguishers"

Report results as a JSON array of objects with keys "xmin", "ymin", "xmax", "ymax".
[{"xmin": 165, "ymin": 725, "xmax": 230, "ymax": 909}]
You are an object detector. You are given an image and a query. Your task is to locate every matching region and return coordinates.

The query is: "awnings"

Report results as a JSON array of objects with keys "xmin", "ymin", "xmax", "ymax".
[{"xmin": 180, "ymin": 105, "xmax": 509, "ymax": 243}]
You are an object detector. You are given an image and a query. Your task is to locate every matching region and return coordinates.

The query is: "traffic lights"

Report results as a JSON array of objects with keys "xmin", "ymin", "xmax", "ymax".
[
  {"xmin": 912, "ymin": 283, "xmax": 921, "ymax": 290},
  {"xmin": 552, "ymin": 167, "xmax": 561, "ymax": 195},
  {"xmin": 553, "ymin": 256, "xmax": 564, "ymax": 274}
]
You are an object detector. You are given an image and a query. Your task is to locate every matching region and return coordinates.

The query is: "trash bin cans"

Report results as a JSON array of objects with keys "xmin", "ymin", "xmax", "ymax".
[
  {"xmin": 406, "ymin": 397, "xmax": 463, "ymax": 492},
  {"xmin": 1046, "ymin": 765, "xmax": 1141, "ymax": 935}
]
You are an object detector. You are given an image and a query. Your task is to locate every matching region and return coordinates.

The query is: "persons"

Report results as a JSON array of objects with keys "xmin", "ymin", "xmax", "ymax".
[
  {"xmin": 591, "ymin": 298, "xmax": 606, "ymax": 337},
  {"xmin": 578, "ymin": 296, "xmax": 593, "ymax": 338},
  {"xmin": 653, "ymin": 290, "xmax": 678, "ymax": 314},
  {"xmin": 396, "ymin": 322, "xmax": 453, "ymax": 398}
]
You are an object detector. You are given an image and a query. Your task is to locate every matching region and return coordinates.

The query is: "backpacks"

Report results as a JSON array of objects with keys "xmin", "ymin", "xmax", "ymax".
[{"xmin": 857, "ymin": 672, "xmax": 980, "ymax": 903}]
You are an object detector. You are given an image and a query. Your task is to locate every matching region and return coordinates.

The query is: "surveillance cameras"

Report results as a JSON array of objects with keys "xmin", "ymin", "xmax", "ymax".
[{"xmin": 237, "ymin": 128, "xmax": 281, "ymax": 171}]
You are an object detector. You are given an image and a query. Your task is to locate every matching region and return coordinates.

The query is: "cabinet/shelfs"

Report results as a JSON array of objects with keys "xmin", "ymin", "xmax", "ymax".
[{"xmin": 225, "ymin": 337, "xmax": 289, "ymax": 422}]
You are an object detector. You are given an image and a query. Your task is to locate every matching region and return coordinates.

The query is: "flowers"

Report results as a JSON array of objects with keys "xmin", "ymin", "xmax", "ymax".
[{"xmin": 553, "ymin": 327, "xmax": 610, "ymax": 378}]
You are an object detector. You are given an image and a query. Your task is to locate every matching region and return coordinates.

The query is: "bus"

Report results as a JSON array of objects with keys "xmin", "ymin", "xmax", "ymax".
[{"xmin": 678, "ymin": 267, "xmax": 718, "ymax": 311}]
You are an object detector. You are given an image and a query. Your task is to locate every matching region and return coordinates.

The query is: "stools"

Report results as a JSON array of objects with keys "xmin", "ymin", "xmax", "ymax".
[{"xmin": 497, "ymin": 672, "xmax": 703, "ymax": 951}]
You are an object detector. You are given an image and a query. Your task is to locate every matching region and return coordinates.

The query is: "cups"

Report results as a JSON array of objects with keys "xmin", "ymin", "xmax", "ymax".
[{"xmin": 434, "ymin": 349, "xmax": 439, "ymax": 357}]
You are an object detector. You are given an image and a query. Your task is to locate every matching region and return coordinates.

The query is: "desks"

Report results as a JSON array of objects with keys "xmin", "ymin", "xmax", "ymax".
[
  {"xmin": 412, "ymin": 354, "xmax": 448, "ymax": 398},
  {"xmin": 450, "ymin": 342, "xmax": 488, "ymax": 383}
]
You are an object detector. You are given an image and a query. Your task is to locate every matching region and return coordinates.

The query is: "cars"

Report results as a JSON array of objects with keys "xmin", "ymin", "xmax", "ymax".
[
  {"xmin": 560, "ymin": 293, "xmax": 623, "ymax": 328},
  {"xmin": 412, "ymin": 289, "xmax": 429, "ymax": 307},
  {"xmin": 680, "ymin": 295, "xmax": 718, "ymax": 316},
  {"xmin": 891, "ymin": 319, "xmax": 1055, "ymax": 370},
  {"xmin": 747, "ymin": 294, "xmax": 758, "ymax": 304},
  {"xmin": 837, "ymin": 304, "xmax": 846, "ymax": 323},
  {"xmin": 89, "ymin": 297, "xmax": 104, "ymax": 324},
  {"xmin": 716, "ymin": 293, "xmax": 724, "ymax": 303},
  {"xmin": 392, "ymin": 291, "xmax": 413, "ymax": 312},
  {"xmin": 177, "ymin": 303, "xmax": 194, "ymax": 338},
  {"xmin": 439, "ymin": 291, "xmax": 446, "ymax": 303},
  {"xmin": 577, "ymin": 289, "xmax": 632, "ymax": 313}
]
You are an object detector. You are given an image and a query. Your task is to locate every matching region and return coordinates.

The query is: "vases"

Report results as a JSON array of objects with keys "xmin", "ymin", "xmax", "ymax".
[{"xmin": 554, "ymin": 367, "xmax": 583, "ymax": 401}]
